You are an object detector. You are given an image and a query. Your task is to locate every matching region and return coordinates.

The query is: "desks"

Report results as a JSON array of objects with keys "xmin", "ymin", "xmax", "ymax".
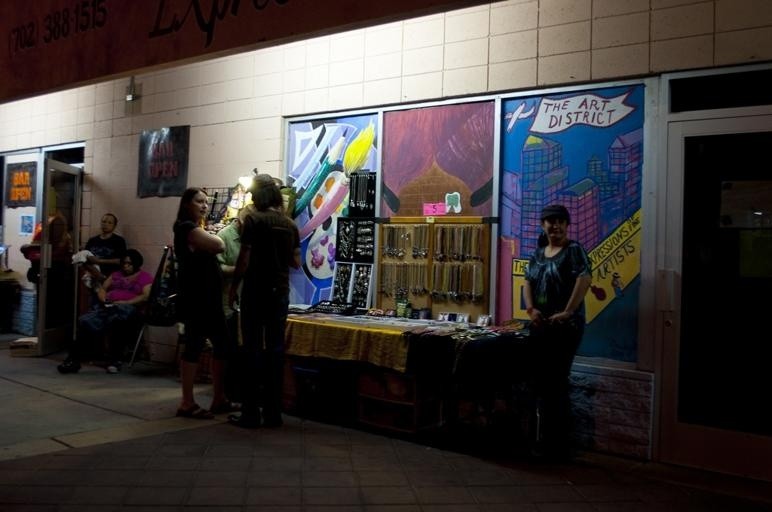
[{"xmin": 236, "ymin": 303, "xmax": 533, "ymax": 448}]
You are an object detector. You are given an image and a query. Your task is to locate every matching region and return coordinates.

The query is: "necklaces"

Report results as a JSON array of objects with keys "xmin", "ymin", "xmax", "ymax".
[
  {"xmin": 349, "ymin": 174, "xmax": 374, "ymax": 210},
  {"xmin": 380, "ymin": 225, "xmax": 485, "ymax": 302}
]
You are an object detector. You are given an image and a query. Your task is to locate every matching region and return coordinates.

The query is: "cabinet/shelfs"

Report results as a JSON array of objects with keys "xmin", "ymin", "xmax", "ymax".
[{"xmin": 355, "ymin": 364, "xmax": 448, "ymax": 442}]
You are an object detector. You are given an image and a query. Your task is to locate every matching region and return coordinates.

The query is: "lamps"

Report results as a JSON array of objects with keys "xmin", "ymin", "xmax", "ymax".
[{"xmin": 125, "ymin": 74, "xmax": 136, "ymax": 101}]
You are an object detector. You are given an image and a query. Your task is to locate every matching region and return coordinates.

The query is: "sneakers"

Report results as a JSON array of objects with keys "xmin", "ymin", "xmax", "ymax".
[
  {"xmin": 106, "ymin": 362, "xmax": 122, "ymax": 374},
  {"xmin": 58, "ymin": 361, "xmax": 80, "ymax": 373},
  {"xmin": 262, "ymin": 417, "xmax": 282, "ymax": 426},
  {"xmin": 228, "ymin": 415, "xmax": 262, "ymax": 429}
]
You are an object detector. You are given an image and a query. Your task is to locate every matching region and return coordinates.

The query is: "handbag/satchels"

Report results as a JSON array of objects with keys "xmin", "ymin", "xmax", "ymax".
[{"xmin": 141, "ymin": 302, "xmax": 178, "ymax": 327}]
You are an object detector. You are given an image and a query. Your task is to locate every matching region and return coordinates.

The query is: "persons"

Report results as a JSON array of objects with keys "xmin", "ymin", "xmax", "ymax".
[
  {"xmin": 83, "ymin": 213, "xmax": 128, "ymax": 309},
  {"xmin": 522, "ymin": 205, "xmax": 592, "ymax": 461},
  {"xmin": 214, "ymin": 204, "xmax": 254, "ymax": 368},
  {"xmin": 25, "ymin": 210, "xmax": 69, "ymax": 327},
  {"xmin": 227, "ymin": 173, "xmax": 301, "ymax": 428},
  {"xmin": 78, "ymin": 245, "xmax": 153, "ymax": 375},
  {"xmin": 172, "ymin": 187, "xmax": 244, "ymax": 420}
]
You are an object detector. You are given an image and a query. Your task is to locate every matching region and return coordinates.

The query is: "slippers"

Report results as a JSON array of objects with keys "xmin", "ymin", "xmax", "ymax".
[
  {"xmin": 210, "ymin": 401, "xmax": 241, "ymax": 414},
  {"xmin": 176, "ymin": 403, "xmax": 214, "ymax": 420}
]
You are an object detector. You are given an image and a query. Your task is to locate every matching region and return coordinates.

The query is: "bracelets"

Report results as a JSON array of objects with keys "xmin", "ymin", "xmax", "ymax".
[
  {"xmin": 336, "ymin": 220, "xmax": 372, "ymax": 306},
  {"xmin": 94, "ymin": 270, "xmax": 101, "ymax": 280},
  {"xmin": 526, "ymin": 306, "xmax": 533, "ymax": 313}
]
